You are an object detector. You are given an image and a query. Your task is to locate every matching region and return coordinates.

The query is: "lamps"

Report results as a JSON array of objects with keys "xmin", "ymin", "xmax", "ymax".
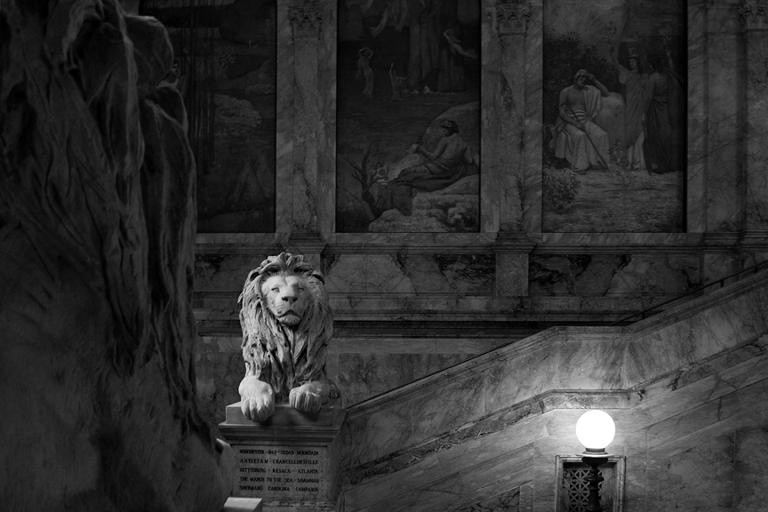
[{"xmin": 576, "ymin": 408, "xmax": 616, "ymax": 510}]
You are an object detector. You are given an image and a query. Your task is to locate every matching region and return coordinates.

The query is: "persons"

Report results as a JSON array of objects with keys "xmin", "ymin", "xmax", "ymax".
[
  {"xmin": 637, "ymin": 55, "xmax": 676, "ymax": 167},
  {"xmin": 374, "ymin": 117, "xmax": 475, "ymax": 213},
  {"xmin": 606, "ymin": 52, "xmax": 647, "ymax": 173},
  {"xmin": 552, "ymin": 68, "xmax": 609, "ymax": 173}
]
